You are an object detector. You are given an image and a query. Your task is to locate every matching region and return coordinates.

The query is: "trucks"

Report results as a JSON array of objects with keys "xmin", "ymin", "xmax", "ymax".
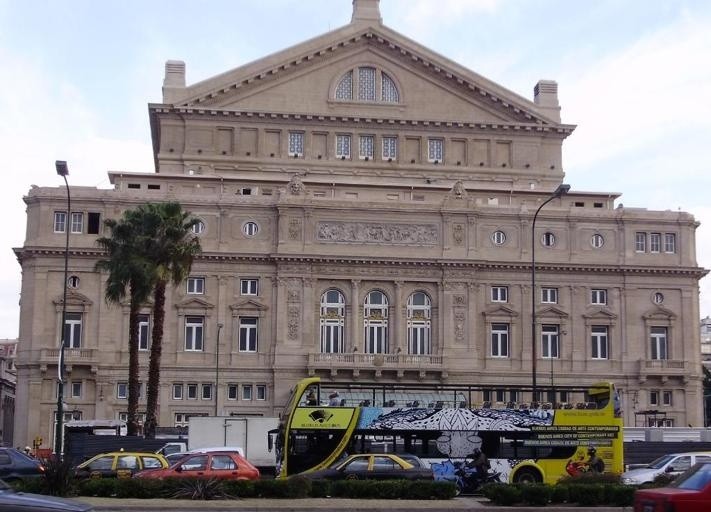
[{"xmin": 155, "ymin": 417, "xmax": 280, "ymax": 475}]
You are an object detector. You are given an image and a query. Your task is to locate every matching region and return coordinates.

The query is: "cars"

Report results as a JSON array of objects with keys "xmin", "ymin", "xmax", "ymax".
[
  {"xmin": 621, "ymin": 449, "xmax": 711, "ymax": 512},
  {"xmin": 303, "ymin": 453, "xmax": 433, "ymax": 480},
  {"xmin": 0, "ymin": 448, "xmax": 259, "ymax": 490},
  {"xmin": 0, "ymin": 479, "xmax": 95, "ymax": 511}
]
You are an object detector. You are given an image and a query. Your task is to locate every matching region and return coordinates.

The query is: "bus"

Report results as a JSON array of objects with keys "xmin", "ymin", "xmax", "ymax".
[{"xmin": 267, "ymin": 377, "xmax": 624, "ymax": 484}]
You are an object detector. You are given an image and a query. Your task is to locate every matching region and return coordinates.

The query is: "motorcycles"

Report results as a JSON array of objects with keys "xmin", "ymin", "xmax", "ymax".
[{"xmin": 451, "ymin": 462, "xmax": 501, "ymax": 497}]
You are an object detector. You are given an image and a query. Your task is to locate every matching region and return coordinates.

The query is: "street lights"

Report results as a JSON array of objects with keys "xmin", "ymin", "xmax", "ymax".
[
  {"xmin": 532, "ymin": 183, "xmax": 570, "ymax": 399},
  {"xmin": 215, "ymin": 323, "xmax": 224, "ymax": 415},
  {"xmin": 54, "ymin": 161, "xmax": 71, "ymax": 455}
]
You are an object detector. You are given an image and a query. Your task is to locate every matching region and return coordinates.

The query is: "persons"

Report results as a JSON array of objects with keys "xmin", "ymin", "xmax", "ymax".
[
  {"xmin": 463, "ymin": 445, "xmax": 492, "ymax": 491},
  {"xmin": 304, "ymin": 393, "xmax": 317, "ymax": 406},
  {"xmin": 576, "ymin": 444, "xmax": 606, "ymax": 475},
  {"xmin": 327, "ymin": 391, "xmax": 342, "ymax": 405}
]
